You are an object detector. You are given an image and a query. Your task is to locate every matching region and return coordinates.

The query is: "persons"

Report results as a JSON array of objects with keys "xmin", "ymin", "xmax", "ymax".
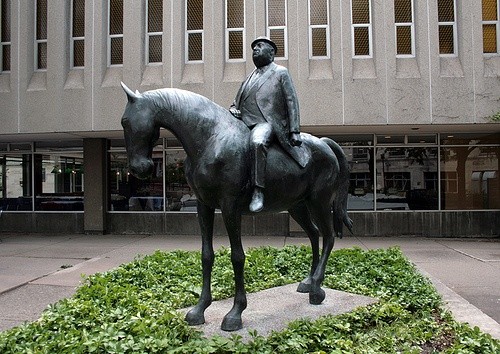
[
  {"xmin": 175, "ymin": 185, "xmax": 193, "ymax": 211},
  {"xmin": 229, "ymin": 36, "xmax": 311, "ymax": 213}
]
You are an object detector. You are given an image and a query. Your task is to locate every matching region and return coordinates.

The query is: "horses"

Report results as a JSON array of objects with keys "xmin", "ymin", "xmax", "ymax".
[{"xmin": 119, "ymin": 78, "xmax": 354, "ymax": 332}]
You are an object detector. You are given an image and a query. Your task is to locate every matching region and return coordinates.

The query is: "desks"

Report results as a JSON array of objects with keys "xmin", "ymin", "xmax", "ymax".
[
  {"xmin": 129, "ymin": 196, "xmax": 168, "ymax": 210},
  {"xmin": 40, "ymin": 201, "xmax": 78, "ymax": 211}
]
